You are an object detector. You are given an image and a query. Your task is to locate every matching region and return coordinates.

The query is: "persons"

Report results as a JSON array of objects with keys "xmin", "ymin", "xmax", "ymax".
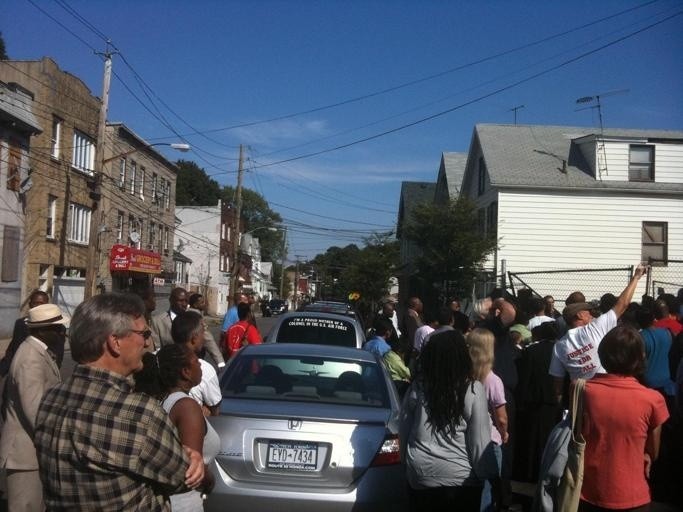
[
  {"xmin": 33, "ymin": 291, "xmax": 204, "ymax": 511},
  {"xmin": 0, "ymin": 303, "xmax": 69, "ymax": 511},
  {"xmin": 0, "ymin": 292, "xmax": 65, "ymax": 420}
]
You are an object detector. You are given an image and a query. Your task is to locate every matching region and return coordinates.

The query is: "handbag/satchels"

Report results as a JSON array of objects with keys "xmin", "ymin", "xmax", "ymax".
[
  {"xmin": 239, "ymin": 324, "xmax": 251, "ymax": 347},
  {"xmin": 531, "ymin": 377, "xmax": 587, "ymax": 512}
]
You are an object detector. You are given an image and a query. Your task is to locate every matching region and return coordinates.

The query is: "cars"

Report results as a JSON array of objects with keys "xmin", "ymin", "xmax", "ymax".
[
  {"xmin": 198, "ymin": 342, "xmax": 409, "ymax": 511},
  {"xmin": 298, "ymin": 296, "xmax": 366, "ymax": 337},
  {"xmin": 261, "ymin": 311, "xmax": 366, "ymax": 349},
  {"xmin": 262, "ymin": 298, "xmax": 288, "ymax": 317}
]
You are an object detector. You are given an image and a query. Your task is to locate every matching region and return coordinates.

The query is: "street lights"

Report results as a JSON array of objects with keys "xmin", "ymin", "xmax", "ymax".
[
  {"xmin": 227, "ymin": 225, "xmax": 277, "ymax": 311},
  {"xmin": 83, "ymin": 143, "xmax": 190, "ymax": 303},
  {"xmin": 278, "ymin": 226, "xmax": 325, "ymax": 312}
]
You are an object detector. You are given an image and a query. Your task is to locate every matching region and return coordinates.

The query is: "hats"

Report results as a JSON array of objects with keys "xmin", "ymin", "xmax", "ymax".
[
  {"xmin": 23, "ymin": 303, "xmax": 67, "ymax": 328},
  {"xmin": 561, "ymin": 302, "xmax": 593, "ymax": 322}
]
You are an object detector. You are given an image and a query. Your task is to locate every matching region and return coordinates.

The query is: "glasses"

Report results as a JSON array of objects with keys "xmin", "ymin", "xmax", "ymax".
[{"xmin": 128, "ymin": 327, "xmax": 152, "ymax": 339}]
